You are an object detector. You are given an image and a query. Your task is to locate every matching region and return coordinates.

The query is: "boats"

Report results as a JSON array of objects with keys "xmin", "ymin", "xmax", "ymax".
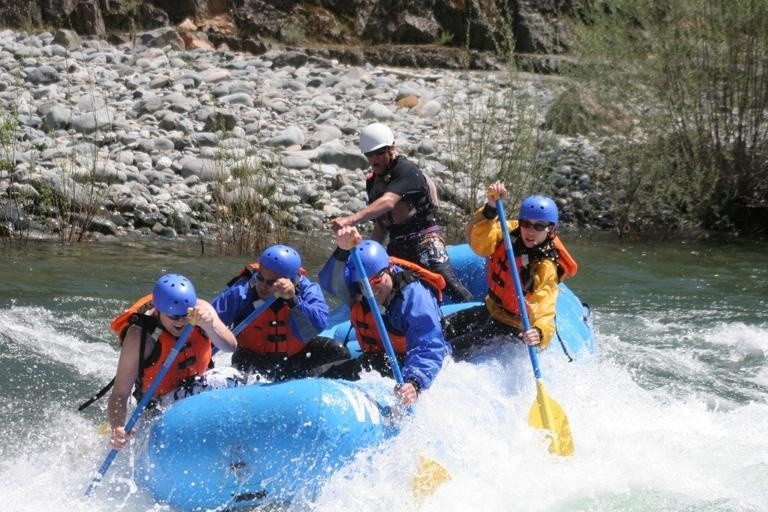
[{"xmin": 127, "ymin": 240, "xmax": 597, "ymax": 507}]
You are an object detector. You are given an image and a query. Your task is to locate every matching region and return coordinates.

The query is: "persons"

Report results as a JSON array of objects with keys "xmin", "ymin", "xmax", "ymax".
[
  {"xmin": 318, "ymin": 225, "xmax": 449, "ymax": 409},
  {"xmin": 329, "ymin": 121, "xmax": 475, "ymax": 305},
  {"xmin": 443, "ymin": 180, "xmax": 560, "ymax": 359},
  {"xmin": 106, "ymin": 271, "xmax": 241, "ymax": 452},
  {"xmin": 210, "ymin": 242, "xmax": 354, "ymax": 382}
]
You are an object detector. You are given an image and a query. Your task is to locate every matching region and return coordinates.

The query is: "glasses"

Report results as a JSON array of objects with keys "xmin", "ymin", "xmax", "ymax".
[
  {"xmin": 167, "ymin": 315, "xmax": 187, "ymax": 320},
  {"xmin": 520, "ymin": 219, "xmax": 551, "ymax": 231},
  {"xmin": 364, "ymin": 146, "xmax": 390, "ymax": 158},
  {"xmin": 369, "ymin": 270, "xmax": 388, "ymax": 285},
  {"xmin": 256, "ymin": 276, "xmax": 278, "ymax": 286}
]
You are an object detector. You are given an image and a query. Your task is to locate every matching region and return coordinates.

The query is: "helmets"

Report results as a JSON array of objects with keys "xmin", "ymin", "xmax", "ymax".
[
  {"xmin": 360, "ymin": 123, "xmax": 395, "ymax": 154},
  {"xmin": 258, "ymin": 244, "xmax": 302, "ymax": 281},
  {"xmin": 153, "ymin": 274, "xmax": 196, "ymax": 316},
  {"xmin": 518, "ymin": 195, "xmax": 558, "ymax": 228},
  {"xmin": 347, "ymin": 241, "xmax": 389, "ymax": 284}
]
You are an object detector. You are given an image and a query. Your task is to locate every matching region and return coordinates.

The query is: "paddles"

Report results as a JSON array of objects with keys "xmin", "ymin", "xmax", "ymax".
[
  {"xmin": 487, "ymin": 188, "xmax": 574, "ymax": 458},
  {"xmin": 340, "ymin": 236, "xmax": 451, "ymax": 508}
]
